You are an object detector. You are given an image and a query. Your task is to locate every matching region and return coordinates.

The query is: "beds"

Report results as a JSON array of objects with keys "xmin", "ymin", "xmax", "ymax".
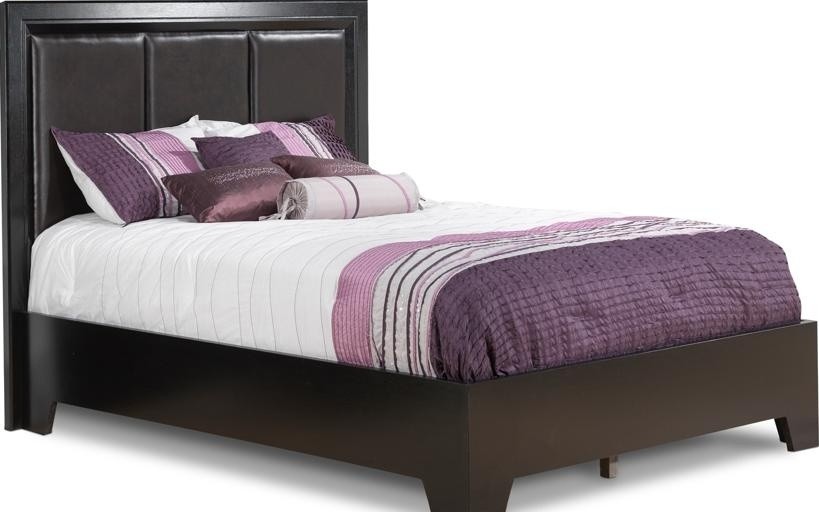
[{"xmin": 0, "ymin": 0, "xmax": 819, "ymax": 512}]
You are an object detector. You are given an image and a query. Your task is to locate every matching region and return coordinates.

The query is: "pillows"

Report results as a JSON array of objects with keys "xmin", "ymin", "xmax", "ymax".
[
  {"xmin": 192, "ymin": 130, "xmax": 291, "ymax": 169},
  {"xmin": 260, "ymin": 171, "xmax": 427, "ymax": 223},
  {"xmin": 160, "ymin": 159, "xmax": 293, "ymax": 224},
  {"xmin": 197, "ymin": 112, "xmax": 361, "ymax": 163},
  {"xmin": 50, "ymin": 114, "xmax": 207, "ymax": 229},
  {"xmin": 270, "ymin": 155, "xmax": 380, "ymax": 178}
]
